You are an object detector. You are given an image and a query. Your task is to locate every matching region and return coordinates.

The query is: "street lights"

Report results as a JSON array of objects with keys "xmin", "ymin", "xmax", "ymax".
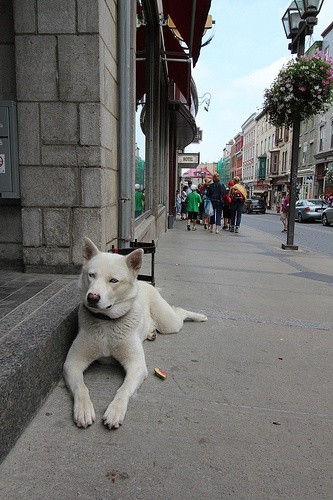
[
  {"xmin": 200, "ymin": 172, "xmax": 204, "ymax": 183},
  {"xmin": 280, "ymin": 0, "xmax": 325, "ymax": 251}
]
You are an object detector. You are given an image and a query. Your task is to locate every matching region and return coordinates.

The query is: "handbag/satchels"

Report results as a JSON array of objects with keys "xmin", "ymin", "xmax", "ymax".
[{"xmin": 204, "ymin": 200, "xmax": 214, "ymax": 216}]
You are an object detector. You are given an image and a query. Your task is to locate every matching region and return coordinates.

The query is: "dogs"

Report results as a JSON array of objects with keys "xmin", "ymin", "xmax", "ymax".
[{"xmin": 63, "ymin": 236, "xmax": 209, "ymax": 430}]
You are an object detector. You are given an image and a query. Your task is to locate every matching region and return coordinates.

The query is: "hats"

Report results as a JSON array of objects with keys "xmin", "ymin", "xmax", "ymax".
[
  {"xmin": 191, "ymin": 185, "xmax": 197, "ymax": 190},
  {"xmin": 229, "ymin": 181, "xmax": 234, "ymax": 186},
  {"xmin": 135, "ymin": 184, "xmax": 141, "ymax": 190}
]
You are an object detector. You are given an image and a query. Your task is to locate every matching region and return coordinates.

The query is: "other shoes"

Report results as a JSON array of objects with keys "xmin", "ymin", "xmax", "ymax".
[
  {"xmin": 235, "ymin": 226, "xmax": 239, "ymax": 232},
  {"xmin": 230, "ymin": 225, "xmax": 234, "ymax": 231},
  {"xmin": 187, "ymin": 224, "xmax": 191, "ymax": 231},
  {"xmin": 204, "ymin": 223, "xmax": 207, "ymax": 229},
  {"xmin": 222, "ymin": 225, "xmax": 229, "ymax": 230},
  {"xmin": 192, "ymin": 227, "xmax": 197, "ymax": 230},
  {"xmin": 282, "ymin": 228, "xmax": 288, "ymax": 232}
]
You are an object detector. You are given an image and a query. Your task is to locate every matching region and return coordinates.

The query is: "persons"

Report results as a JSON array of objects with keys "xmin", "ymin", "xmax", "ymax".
[
  {"xmin": 280, "ymin": 185, "xmax": 290, "ymax": 233},
  {"xmin": 135, "ymin": 184, "xmax": 145, "ymax": 218},
  {"xmin": 174, "ymin": 173, "xmax": 246, "ymax": 234},
  {"xmin": 317, "ymin": 192, "xmax": 333, "ymax": 208}
]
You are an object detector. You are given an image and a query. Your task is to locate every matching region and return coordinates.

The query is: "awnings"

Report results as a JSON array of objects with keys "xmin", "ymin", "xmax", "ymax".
[
  {"xmin": 164, "ymin": 0, "xmax": 211, "ymax": 68},
  {"xmin": 163, "ymin": 24, "xmax": 192, "ymax": 112}
]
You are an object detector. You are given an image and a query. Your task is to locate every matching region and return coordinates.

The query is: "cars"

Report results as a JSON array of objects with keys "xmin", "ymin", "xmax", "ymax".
[
  {"xmin": 242, "ymin": 199, "xmax": 266, "ymax": 214},
  {"xmin": 321, "ymin": 206, "xmax": 333, "ymax": 227},
  {"xmin": 295, "ymin": 199, "xmax": 328, "ymax": 223}
]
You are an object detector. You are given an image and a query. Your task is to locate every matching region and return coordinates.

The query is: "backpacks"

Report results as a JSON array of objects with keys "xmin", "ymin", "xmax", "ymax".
[{"xmin": 231, "ymin": 188, "xmax": 243, "ymax": 203}]
